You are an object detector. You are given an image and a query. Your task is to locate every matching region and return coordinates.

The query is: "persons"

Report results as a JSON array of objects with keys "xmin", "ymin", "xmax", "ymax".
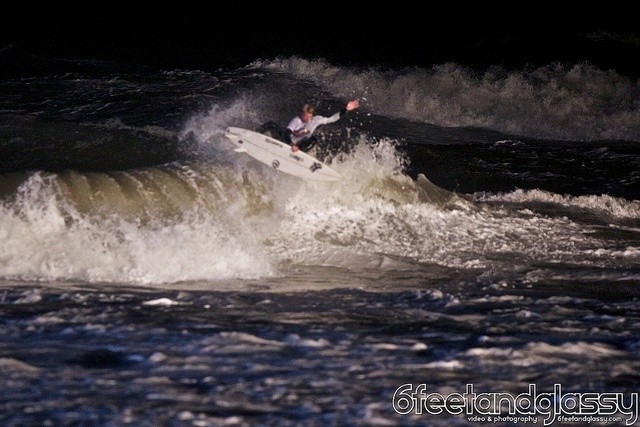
[{"xmin": 254, "ymin": 100, "xmax": 360, "ymax": 153}]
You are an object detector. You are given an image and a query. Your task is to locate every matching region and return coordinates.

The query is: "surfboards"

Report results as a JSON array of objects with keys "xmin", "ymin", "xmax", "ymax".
[{"xmin": 222, "ymin": 126, "xmax": 343, "ymax": 183}]
